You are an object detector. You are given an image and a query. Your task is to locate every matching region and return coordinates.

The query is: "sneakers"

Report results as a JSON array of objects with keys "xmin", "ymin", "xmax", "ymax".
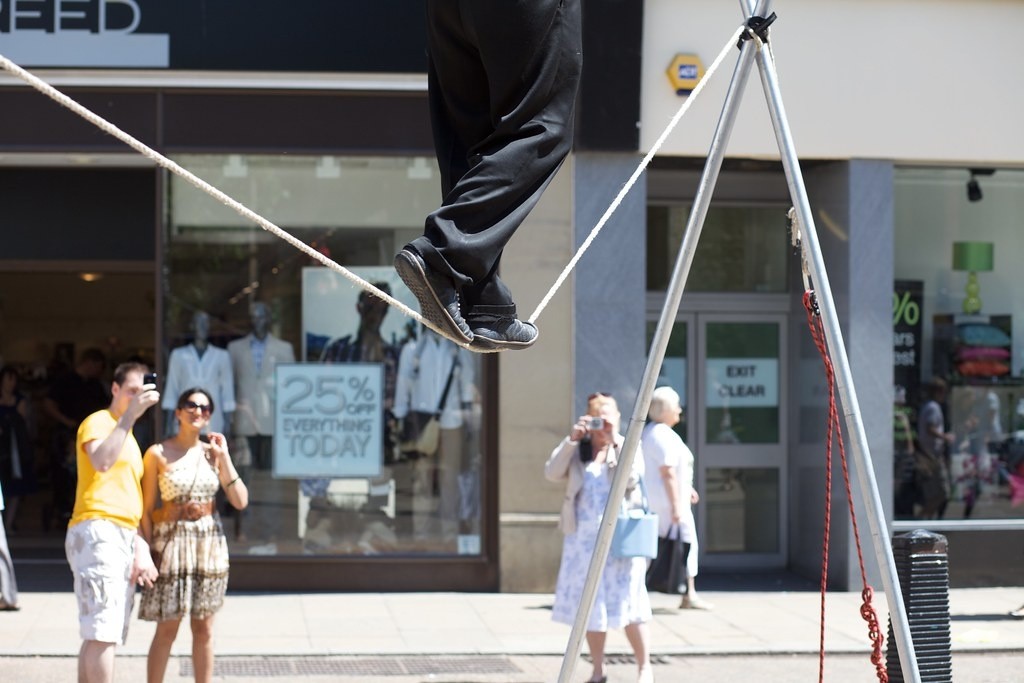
[
  {"xmin": 394, "ymin": 247, "xmax": 475, "ymax": 346},
  {"xmin": 471, "ymin": 318, "xmax": 538, "ymax": 350}
]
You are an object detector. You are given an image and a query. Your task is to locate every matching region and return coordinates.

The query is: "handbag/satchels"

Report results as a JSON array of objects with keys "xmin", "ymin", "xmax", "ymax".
[
  {"xmin": 398, "ymin": 409, "xmax": 441, "ymax": 459},
  {"xmin": 151, "ymin": 549, "xmax": 163, "ymax": 584},
  {"xmin": 644, "ymin": 522, "xmax": 690, "ymax": 597}
]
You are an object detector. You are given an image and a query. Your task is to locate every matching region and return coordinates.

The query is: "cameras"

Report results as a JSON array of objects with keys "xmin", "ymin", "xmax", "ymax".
[{"xmin": 584, "ymin": 417, "xmax": 605, "ymax": 431}]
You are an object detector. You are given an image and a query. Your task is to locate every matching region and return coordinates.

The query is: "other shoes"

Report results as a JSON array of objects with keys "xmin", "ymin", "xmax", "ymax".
[
  {"xmin": 585, "ymin": 676, "xmax": 607, "ymax": 683},
  {"xmin": 0, "ymin": 599, "xmax": 21, "ymax": 612}
]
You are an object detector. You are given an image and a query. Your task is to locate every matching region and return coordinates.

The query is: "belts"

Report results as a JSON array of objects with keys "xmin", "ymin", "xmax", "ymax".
[{"xmin": 150, "ymin": 499, "xmax": 216, "ymax": 524}]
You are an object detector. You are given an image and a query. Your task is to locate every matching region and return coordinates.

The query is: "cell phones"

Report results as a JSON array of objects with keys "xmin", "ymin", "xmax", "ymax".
[{"xmin": 144, "ymin": 373, "xmax": 157, "ymax": 392}]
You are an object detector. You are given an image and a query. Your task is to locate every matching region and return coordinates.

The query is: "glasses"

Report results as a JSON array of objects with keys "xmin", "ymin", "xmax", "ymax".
[
  {"xmin": 182, "ymin": 401, "xmax": 213, "ymax": 421},
  {"xmin": 587, "ymin": 392, "xmax": 611, "ymax": 407}
]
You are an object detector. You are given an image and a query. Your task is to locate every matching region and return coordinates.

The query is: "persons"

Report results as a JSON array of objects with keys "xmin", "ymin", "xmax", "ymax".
[
  {"xmin": 395, "ymin": 0, "xmax": 582, "ymax": 351},
  {"xmin": 163, "ymin": 302, "xmax": 296, "ymax": 473},
  {"xmin": 0, "ymin": 480, "xmax": 17, "ymax": 611},
  {"xmin": 0, "ymin": 347, "xmax": 153, "ymax": 536},
  {"xmin": 66, "ymin": 361, "xmax": 159, "ymax": 683},
  {"xmin": 137, "ymin": 387, "xmax": 248, "ymax": 683},
  {"xmin": 894, "ymin": 376, "xmax": 1024, "ymax": 520},
  {"xmin": 321, "ymin": 282, "xmax": 397, "ymax": 433},
  {"xmin": 643, "ymin": 387, "xmax": 713, "ymax": 610},
  {"xmin": 216, "ymin": 383, "xmax": 261, "ymax": 545},
  {"xmin": 393, "ymin": 325, "xmax": 476, "ymax": 554},
  {"xmin": 544, "ymin": 393, "xmax": 658, "ymax": 683}
]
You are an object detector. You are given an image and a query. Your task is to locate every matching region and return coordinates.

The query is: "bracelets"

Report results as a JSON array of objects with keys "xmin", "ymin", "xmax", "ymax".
[{"xmin": 225, "ymin": 476, "xmax": 241, "ymax": 492}]
